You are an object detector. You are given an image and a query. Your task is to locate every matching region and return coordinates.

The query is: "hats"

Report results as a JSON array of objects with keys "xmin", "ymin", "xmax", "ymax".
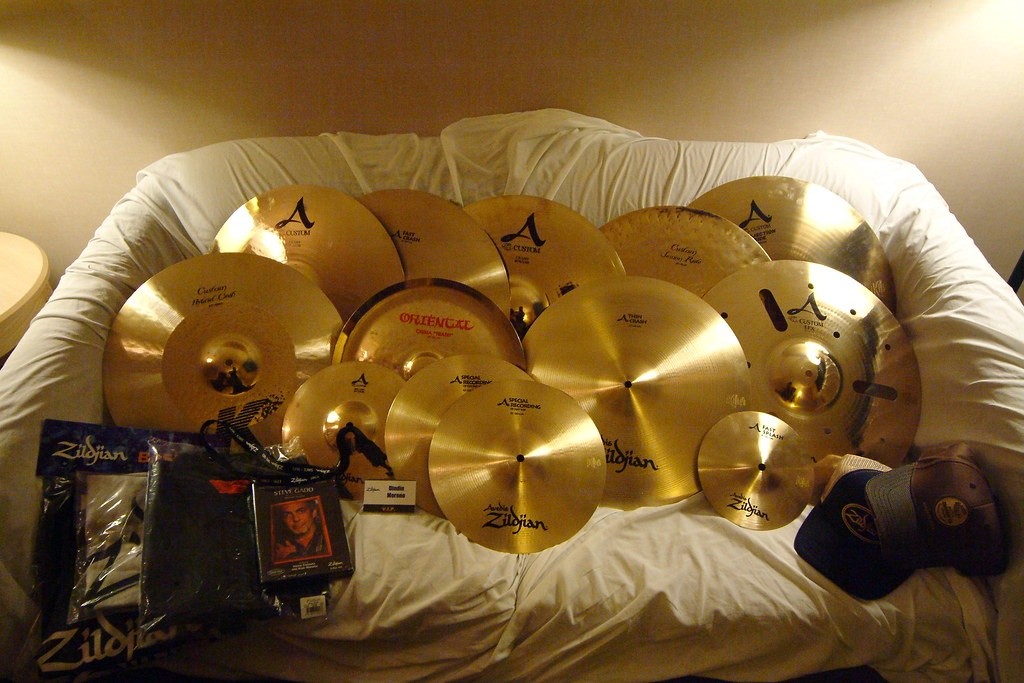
[
  {"xmin": 862, "ymin": 439, "xmax": 1008, "ymax": 578},
  {"xmin": 793, "ymin": 468, "xmax": 917, "ymax": 601},
  {"xmin": 817, "ymin": 452, "xmax": 894, "ymax": 504}
]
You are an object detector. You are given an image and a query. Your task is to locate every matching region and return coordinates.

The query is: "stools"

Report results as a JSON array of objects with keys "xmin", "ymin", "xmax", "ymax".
[{"xmin": 0, "ymin": 223, "xmax": 51, "ymax": 362}]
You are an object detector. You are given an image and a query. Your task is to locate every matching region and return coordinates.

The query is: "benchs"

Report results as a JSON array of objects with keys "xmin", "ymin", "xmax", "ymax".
[{"xmin": 0, "ymin": 107, "xmax": 1024, "ymax": 683}]
